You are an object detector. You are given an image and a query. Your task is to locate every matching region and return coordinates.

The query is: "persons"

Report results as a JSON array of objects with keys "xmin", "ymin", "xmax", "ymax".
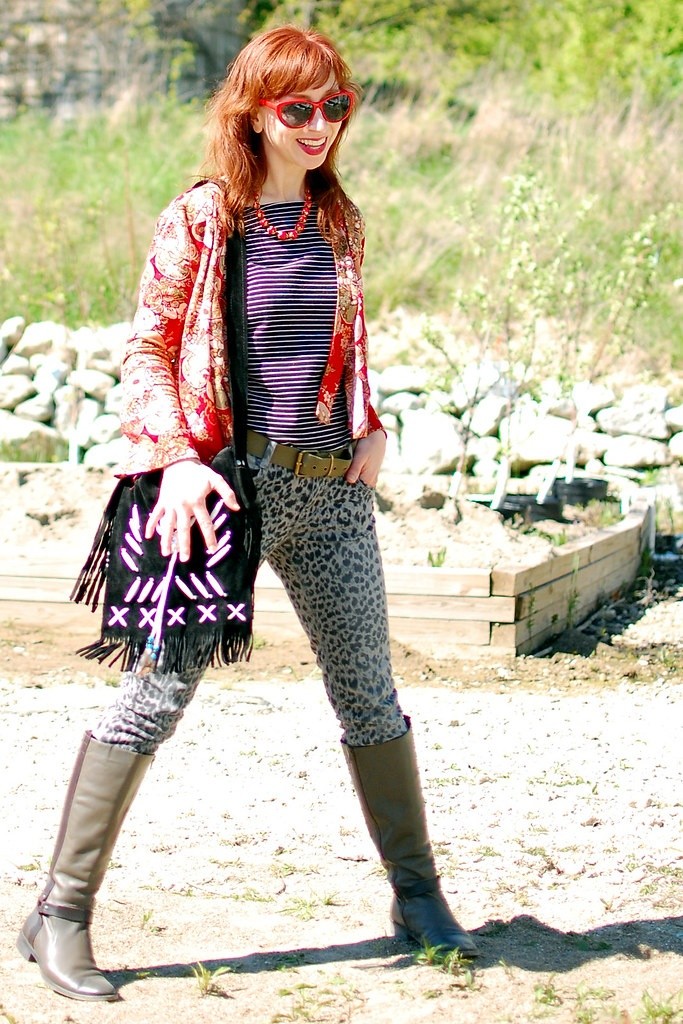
[{"xmin": 17, "ymin": 26, "xmax": 479, "ymax": 1002}]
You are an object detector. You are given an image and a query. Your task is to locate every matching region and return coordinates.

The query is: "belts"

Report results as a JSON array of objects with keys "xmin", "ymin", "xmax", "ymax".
[{"xmin": 249, "ymin": 430, "xmax": 352, "ymax": 476}]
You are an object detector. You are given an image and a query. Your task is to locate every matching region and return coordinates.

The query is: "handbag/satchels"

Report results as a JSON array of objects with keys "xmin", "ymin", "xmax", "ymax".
[{"xmin": 69, "ymin": 447, "xmax": 263, "ymax": 667}]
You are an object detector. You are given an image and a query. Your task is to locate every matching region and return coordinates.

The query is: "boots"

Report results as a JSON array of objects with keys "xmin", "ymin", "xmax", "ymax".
[
  {"xmin": 16, "ymin": 729, "xmax": 153, "ymax": 1001},
  {"xmin": 340, "ymin": 716, "xmax": 478, "ymax": 958}
]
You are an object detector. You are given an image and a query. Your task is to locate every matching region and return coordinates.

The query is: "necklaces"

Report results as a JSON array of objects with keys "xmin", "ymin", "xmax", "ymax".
[{"xmin": 253, "ymin": 181, "xmax": 311, "ymax": 240}]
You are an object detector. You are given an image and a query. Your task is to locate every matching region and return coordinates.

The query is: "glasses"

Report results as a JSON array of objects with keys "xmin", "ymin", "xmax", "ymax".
[{"xmin": 255, "ymin": 87, "xmax": 354, "ymax": 128}]
